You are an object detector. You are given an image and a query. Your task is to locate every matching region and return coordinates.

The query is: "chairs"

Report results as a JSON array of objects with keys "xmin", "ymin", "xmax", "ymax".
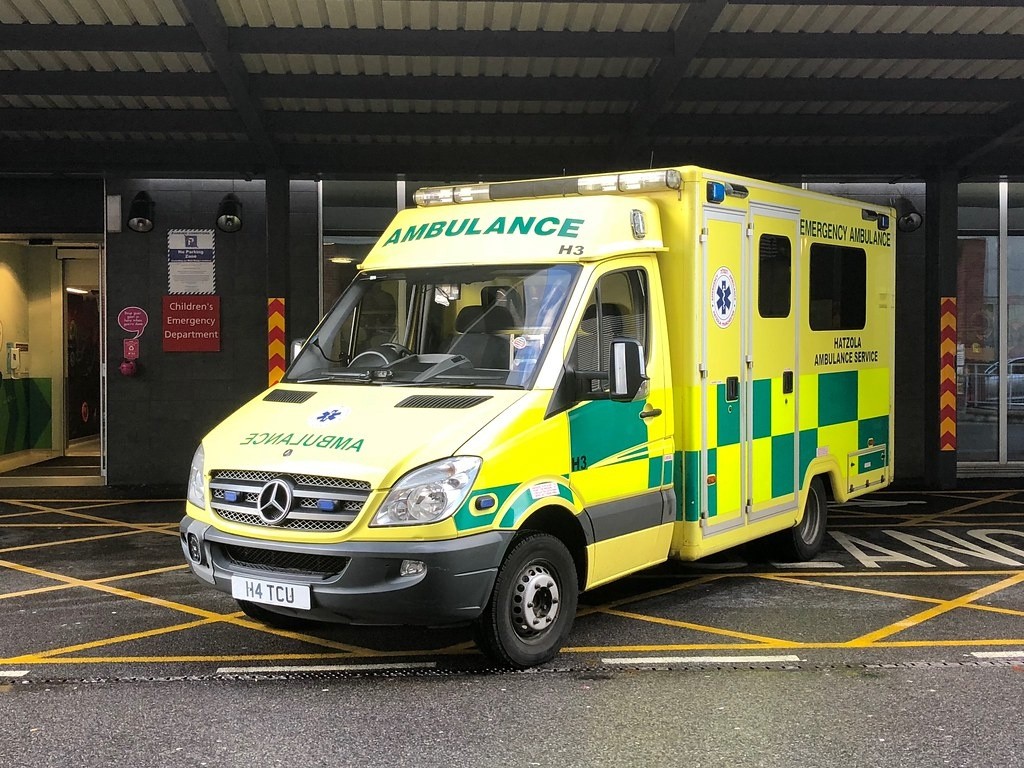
[
  {"xmin": 449, "ymin": 305, "xmax": 518, "ymax": 369},
  {"xmin": 567, "ymin": 302, "xmax": 631, "ymax": 388}
]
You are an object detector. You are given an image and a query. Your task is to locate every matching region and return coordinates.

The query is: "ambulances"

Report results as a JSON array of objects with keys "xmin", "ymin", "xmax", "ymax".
[{"xmin": 178, "ymin": 165, "xmax": 899, "ymax": 666}]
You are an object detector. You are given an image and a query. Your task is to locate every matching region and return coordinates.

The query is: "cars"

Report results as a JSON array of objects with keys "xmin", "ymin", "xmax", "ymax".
[{"xmin": 975, "ymin": 358, "xmax": 1024, "ymax": 401}]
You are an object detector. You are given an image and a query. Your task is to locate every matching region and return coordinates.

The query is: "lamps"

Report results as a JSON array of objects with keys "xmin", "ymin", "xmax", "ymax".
[
  {"xmin": 126, "ymin": 190, "xmax": 156, "ymax": 233},
  {"xmin": 892, "ymin": 198, "xmax": 923, "ymax": 233},
  {"xmin": 216, "ymin": 193, "xmax": 244, "ymax": 233}
]
